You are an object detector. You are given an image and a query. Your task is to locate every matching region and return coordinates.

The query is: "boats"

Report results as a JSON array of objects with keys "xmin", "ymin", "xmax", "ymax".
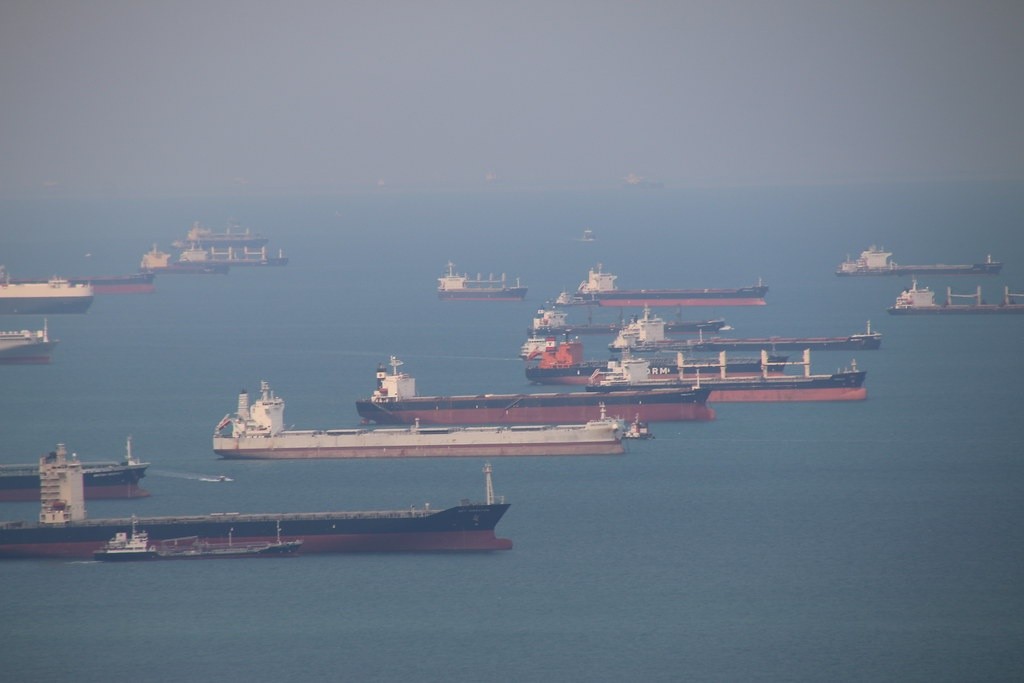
[
  {"xmin": 435, "ymin": 260, "xmax": 528, "ymax": 301},
  {"xmin": 0, "ymin": 312, "xmax": 61, "ymax": 358},
  {"xmin": 519, "ymin": 296, "xmax": 884, "ymax": 402},
  {"xmin": 0, "ymin": 221, "xmax": 289, "ymax": 316},
  {"xmin": 355, "ymin": 354, "xmax": 717, "ymax": 424},
  {"xmin": 0, "ymin": 434, "xmax": 154, "ymax": 500},
  {"xmin": 0, "ymin": 442, "xmax": 516, "ymax": 560},
  {"xmin": 885, "ymin": 277, "xmax": 1024, "ymax": 316},
  {"xmin": 213, "ymin": 380, "xmax": 628, "ymax": 460},
  {"xmin": 543, "ymin": 262, "xmax": 770, "ymax": 307},
  {"xmin": 835, "ymin": 243, "xmax": 1007, "ymax": 277}
]
